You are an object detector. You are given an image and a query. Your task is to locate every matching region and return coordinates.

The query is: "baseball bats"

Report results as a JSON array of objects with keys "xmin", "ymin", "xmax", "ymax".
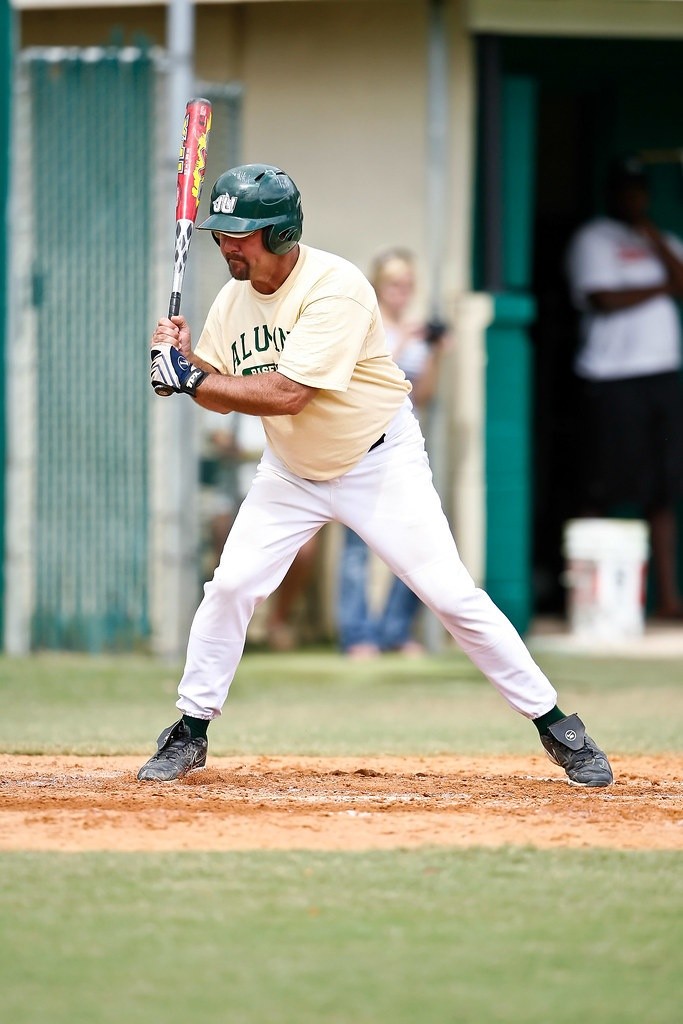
[{"xmin": 154, "ymin": 97, "xmax": 214, "ymax": 398}]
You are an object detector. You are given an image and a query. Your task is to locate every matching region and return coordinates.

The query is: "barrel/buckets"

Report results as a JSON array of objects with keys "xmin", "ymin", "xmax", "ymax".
[{"xmin": 562, "ymin": 518, "xmax": 650, "ymax": 642}]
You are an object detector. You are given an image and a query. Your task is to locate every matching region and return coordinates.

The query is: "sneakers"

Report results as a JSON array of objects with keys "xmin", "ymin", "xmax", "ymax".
[
  {"xmin": 137, "ymin": 719, "xmax": 208, "ymax": 783},
  {"xmin": 540, "ymin": 713, "xmax": 613, "ymax": 788}
]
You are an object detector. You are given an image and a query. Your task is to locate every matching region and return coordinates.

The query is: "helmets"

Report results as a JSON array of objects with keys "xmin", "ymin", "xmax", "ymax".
[{"xmin": 195, "ymin": 163, "xmax": 304, "ymax": 256}]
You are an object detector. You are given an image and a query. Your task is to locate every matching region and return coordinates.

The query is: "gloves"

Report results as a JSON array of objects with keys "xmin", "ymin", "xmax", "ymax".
[{"xmin": 150, "ymin": 344, "xmax": 210, "ymax": 398}]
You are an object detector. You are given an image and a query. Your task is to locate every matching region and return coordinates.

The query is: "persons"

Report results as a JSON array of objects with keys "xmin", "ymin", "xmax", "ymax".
[
  {"xmin": 563, "ymin": 155, "xmax": 683, "ymax": 628},
  {"xmin": 333, "ymin": 247, "xmax": 448, "ymax": 661},
  {"xmin": 137, "ymin": 165, "xmax": 613, "ymax": 787}
]
